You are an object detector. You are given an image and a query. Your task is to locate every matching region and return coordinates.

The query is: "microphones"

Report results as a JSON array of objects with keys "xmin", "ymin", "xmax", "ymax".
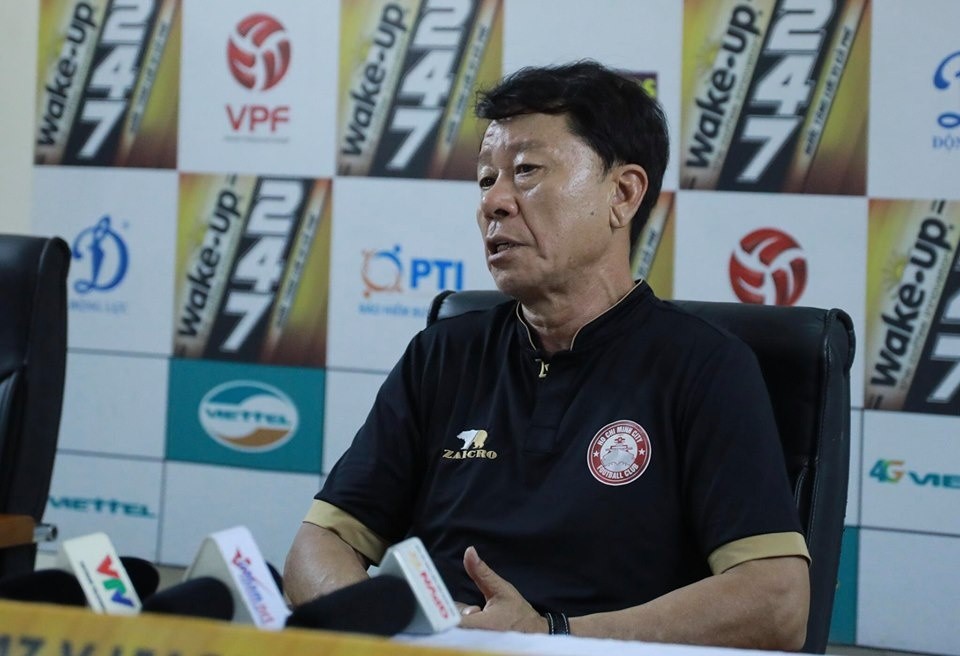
[{"xmin": 13, "ymin": 531, "xmax": 459, "ymax": 640}]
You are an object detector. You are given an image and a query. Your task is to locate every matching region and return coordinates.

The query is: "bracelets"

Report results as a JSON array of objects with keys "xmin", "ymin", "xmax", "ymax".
[{"xmin": 545, "ymin": 612, "xmax": 571, "ymax": 636}]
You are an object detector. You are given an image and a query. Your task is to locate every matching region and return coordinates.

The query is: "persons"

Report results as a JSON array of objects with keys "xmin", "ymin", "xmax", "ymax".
[{"xmin": 281, "ymin": 57, "xmax": 815, "ymax": 653}]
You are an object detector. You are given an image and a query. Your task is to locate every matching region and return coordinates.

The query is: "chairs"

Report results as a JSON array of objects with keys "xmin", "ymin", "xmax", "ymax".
[
  {"xmin": 424, "ymin": 287, "xmax": 860, "ymax": 656},
  {"xmin": 0, "ymin": 231, "xmax": 73, "ymax": 587}
]
